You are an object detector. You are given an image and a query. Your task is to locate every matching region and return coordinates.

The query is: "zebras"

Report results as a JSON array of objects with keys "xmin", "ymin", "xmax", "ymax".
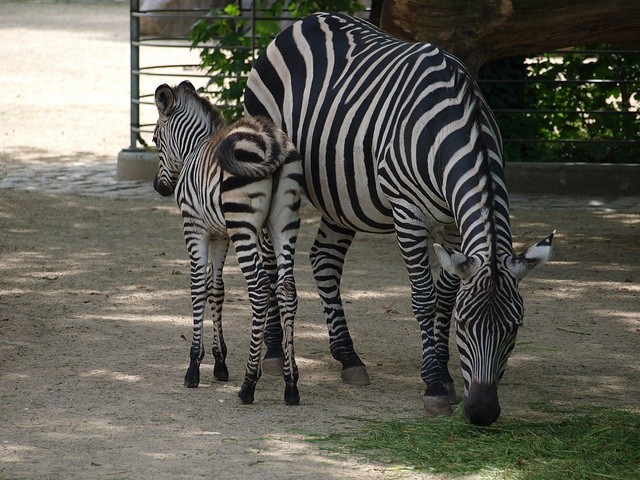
[
  {"xmin": 152, "ymin": 79, "xmax": 300, "ymax": 405},
  {"xmin": 244, "ymin": 10, "xmax": 556, "ymax": 431}
]
